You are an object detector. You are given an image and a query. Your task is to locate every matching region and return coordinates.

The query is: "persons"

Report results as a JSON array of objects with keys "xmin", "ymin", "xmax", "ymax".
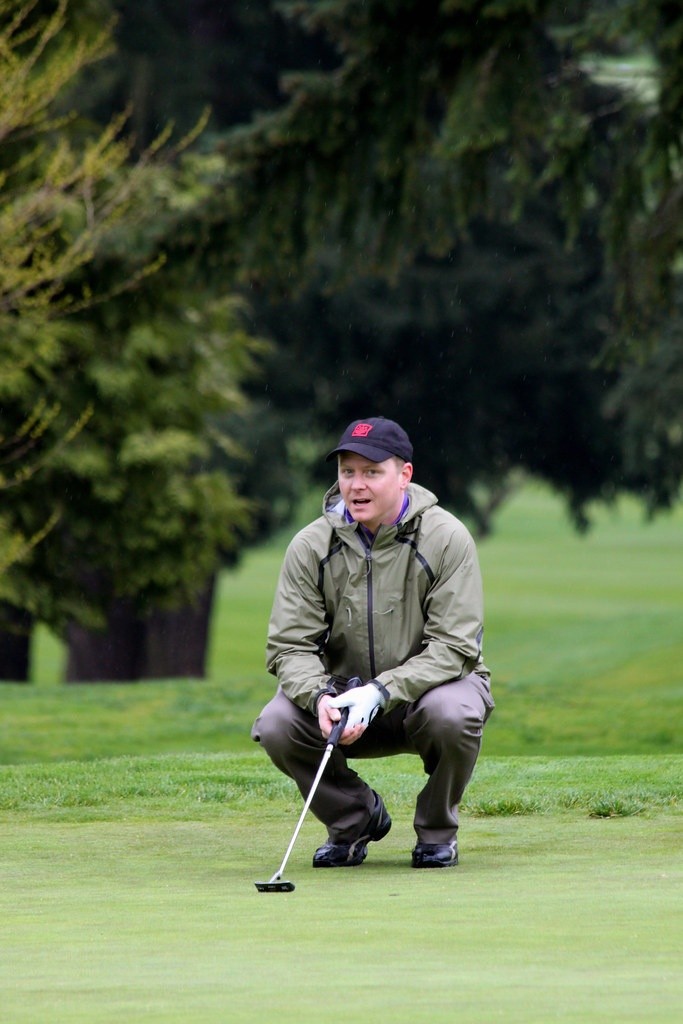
[{"xmin": 250, "ymin": 418, "xmax": 500, "ymax": 871}]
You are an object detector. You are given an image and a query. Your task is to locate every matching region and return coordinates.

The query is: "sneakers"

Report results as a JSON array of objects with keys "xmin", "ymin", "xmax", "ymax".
[
  {"xmin": 313, "ymin": 791, "xmax": 392, "ymax": 865},
  {"xmin": 412, "ymin": 835, "xmax": 459, "ymax": 867}
]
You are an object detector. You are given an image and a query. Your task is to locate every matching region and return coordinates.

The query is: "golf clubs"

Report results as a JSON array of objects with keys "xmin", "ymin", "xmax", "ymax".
[{"xmin": 255, "ymin": 677, "xmax": 362, "ymax": 893}]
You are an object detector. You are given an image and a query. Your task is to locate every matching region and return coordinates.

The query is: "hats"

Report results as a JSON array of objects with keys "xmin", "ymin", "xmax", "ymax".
[{"xmin": 325, "ymin": 416, "xmax": 418, "ymax": 460}]
